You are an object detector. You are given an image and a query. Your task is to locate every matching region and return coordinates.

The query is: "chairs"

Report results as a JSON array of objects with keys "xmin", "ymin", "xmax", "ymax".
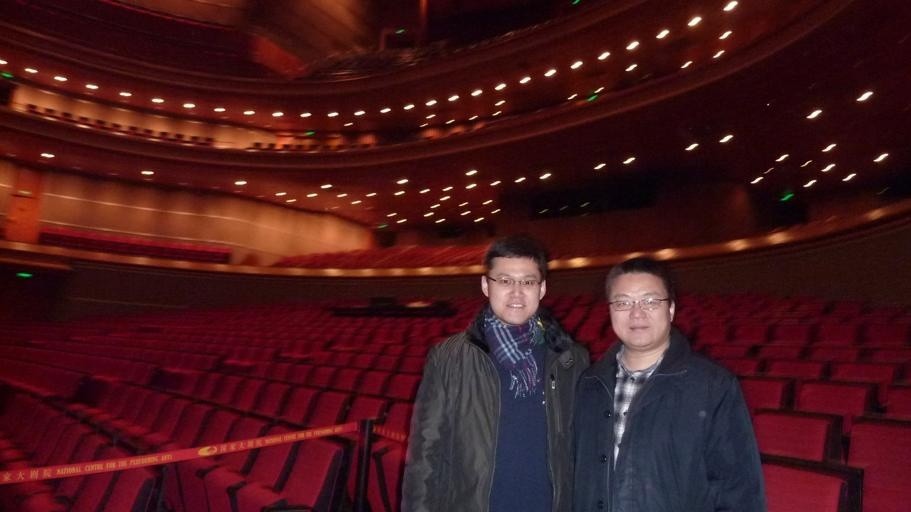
[{"xmin": 0, "ymin": 224, "xmax": 911, "ymax": 512}]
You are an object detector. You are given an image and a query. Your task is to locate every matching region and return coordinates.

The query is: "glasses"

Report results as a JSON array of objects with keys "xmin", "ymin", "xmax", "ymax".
[
  {"xmin": 485, "ymin": 275, "xmax": 544, "ymax": 289},
  {"xmin": 608, "ymin": 297, "xmax": 672, "ymax": 312}
]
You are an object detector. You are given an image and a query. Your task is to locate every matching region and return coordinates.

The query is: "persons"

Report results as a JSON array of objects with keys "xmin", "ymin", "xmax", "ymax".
[
  {"xmin": 568, "ymin": 257, "xmax": 767, "ymax": 510},
  {"xmin": 402, "ymin": 233, "xmax": 594, "ymax": 510}
]
